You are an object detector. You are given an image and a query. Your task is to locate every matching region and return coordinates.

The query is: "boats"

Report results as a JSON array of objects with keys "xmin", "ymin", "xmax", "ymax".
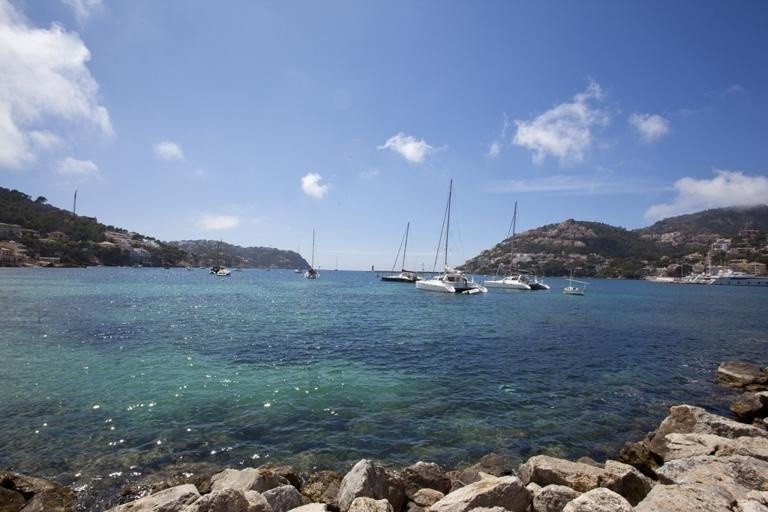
[
  {"xmin": 233, "ymin": 268, "xmax": 239, "ymax": 271},
  {"xmin": 294, "ymin": 269, "xmax": 305, "ymax": 273},
  {"xmin": 186, "ymin": 266, "xmax": 193, "ymax": 271},
  {"xmin": 200, "ymin": 266, "xmax": 205, "ymax": 269},
  {"xmin": 562, "ymin": 269, "xmax": 589, "ymax": 295}
]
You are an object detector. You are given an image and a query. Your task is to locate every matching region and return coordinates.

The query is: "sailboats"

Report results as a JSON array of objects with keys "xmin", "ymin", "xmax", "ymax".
[
  {"xmin": 216, "ymin": 238, "xmax": 231, "ymax": 276},
  {"xmin": 304, "ymin": 228, "xmax": 321, "ymax": 280},
  {"xmin": 482, "ymin": 199, "xmax": 550, "ymax": 291},
  {"xmin": 382, "ymin": 222, "xmax": 423, "ymax": 283},
  {"xmin": 370, "ymin": 265, "xmax": 376, "ymax": 273},
  {"xmin": 209, "ymin": 240, "xmax": 223, "ymax": 274},
  {"xmin": 334, "ymin": 256, "xmax": 338, "ymax": 271},
  {"xmin": 415, "ymin": 178, "xmax": 488, "ymax": 294}
]
